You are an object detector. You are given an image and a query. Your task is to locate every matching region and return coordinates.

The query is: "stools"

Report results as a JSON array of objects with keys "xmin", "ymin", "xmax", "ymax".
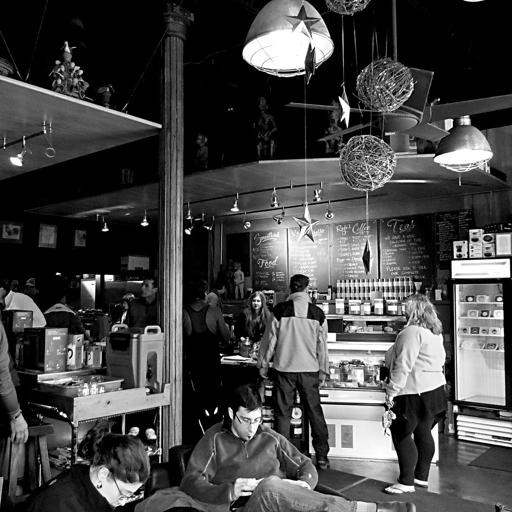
[{"xmin": 0, "ymin": 413, "xmax": 53, "ymax": 507}]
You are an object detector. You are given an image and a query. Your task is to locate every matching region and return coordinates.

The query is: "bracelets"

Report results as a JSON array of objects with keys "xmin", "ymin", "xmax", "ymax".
[{"xmin": 9, "ymin": 409, "xmax": 22, "ymax": 420}]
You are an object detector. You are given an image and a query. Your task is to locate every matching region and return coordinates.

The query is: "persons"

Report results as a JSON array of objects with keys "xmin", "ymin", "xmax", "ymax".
[
  {"xmin": 236, "ymin": 290, "xmax": 278, "ymax": 343},
  {"xmin": 126, "ymin": 278, "xmax": 160, "ymax": 329},
  {"xmin": 205, "ymin": 281, "xmax": 227, "ymax": 309},
  {"xmin": 120, "ymin": 293, "xmax": 135, "ymax": 323},
  {"xmin": 183, "ymin": 281, "xmax": 232, "ymax": 397},
  {"xmin": 181, "ymin": 385, "xmax": 415, "ymax": 512},
  {"xmin": 385, "ymin": 292, "xmax": 447, "ymax": 494},
  {"xmin": 256, "ymin": 274, "xmax": 330, "ymax": 470},
  {"xmin": 0, "ymin": 287, "xmax": 46, "ymax": 332},
  {"xmin": 0, "ymin": 320, "xmax": 30, "ymax": 512},
  {"xmin": 43, "ymin": 290, "xmax": 95, "ymax": 347},
  {"xmin": 23, "ymin": 434, "xmax": 149, "ymax": 511}
]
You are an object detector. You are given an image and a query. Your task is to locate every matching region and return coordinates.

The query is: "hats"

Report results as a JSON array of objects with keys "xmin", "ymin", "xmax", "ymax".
[{"xmin": 290, "ymin": 274, "xmax": 310, "ymax": 293}]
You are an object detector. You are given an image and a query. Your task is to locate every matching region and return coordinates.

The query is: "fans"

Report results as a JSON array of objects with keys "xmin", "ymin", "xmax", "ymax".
[{"xmin": 288, "ymin": 0, "xmax": 512, "ymax": 190}]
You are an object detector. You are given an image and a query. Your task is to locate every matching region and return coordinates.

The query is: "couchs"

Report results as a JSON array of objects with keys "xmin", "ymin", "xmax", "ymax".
[{"xmin": 147, "ymin": 444, "xmax": 352, "ymax": 512}]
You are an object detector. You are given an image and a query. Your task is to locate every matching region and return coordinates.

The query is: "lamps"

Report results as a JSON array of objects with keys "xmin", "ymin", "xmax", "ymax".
[
  {"xmin": 229, "ymin": 193, "xmax": 239, "ymax": 212},
  {"xmin": 241, "ymin": 212, "xmax": 251, "ymax": 230},
  {"xmin": 273, "ymin": 208, "xmax": 285, "ymax": 224},
  {"xmin": 325, "ymin": 202, "xmax": 334, "ymax": 220},
  {"xmin": 312, "ymin": 183, "xmax": 324, "ymax": 202},
  {"xmin": 141, "ymin": 209, "xmax": 150, "ymax": 228},
  {"xmin": 238, "ymin": 0, "xmax": 334, "ymax": 77},
  {"xmin": 101, "ymin": 215, "xmax": 110, "ymax": 233},
  {"xmin": 184, "ymin": 224, "xmax": 196, "ymax": 235},
  {"xmin": 271, "ymin": 188, "xmax": 280, "ymax": 207},
  {"xmin": 433, "ymin": 123, "xmax": 494, "ymax": 173},
  {"xmin": 9, "ymin": 149, "xmax": 26, "ymax": 167},
  {"xmin": 185, "ymin": 201, "xmax": 193, "ymax": 220}
]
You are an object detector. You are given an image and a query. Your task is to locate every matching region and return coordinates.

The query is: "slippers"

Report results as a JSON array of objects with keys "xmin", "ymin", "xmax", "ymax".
[
  {"xmin": 384, "ymin": 483, "xmax": 414, "ymax": 493},
  {"xmin": 413, "ymin": 476, "xmax": 429, "ymax": 487}
]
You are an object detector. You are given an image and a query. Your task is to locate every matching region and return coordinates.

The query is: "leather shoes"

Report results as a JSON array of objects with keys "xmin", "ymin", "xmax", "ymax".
[{"xmin": 375, "ymin": 500, "xmax": 416, "ymax": 512}]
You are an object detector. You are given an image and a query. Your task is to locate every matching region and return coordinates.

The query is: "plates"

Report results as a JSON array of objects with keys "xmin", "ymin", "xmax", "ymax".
[{"xmin": 37, "ymin": 375, "xmax": 126, "ymax": 396}]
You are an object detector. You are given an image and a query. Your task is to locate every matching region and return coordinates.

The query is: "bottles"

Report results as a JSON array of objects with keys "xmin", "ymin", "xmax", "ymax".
[
  {"xmin": 401, "ymin": 302, "xmax": 407, "ymax": 317},
  {"xmin": 373, "ymin": 299, "xmax": 384, "ymax": 315},
  {"xmin": 386, "ymin": 299, "xmax": 398, "ymax": 316},
  {"xmin": 348, "ymin": 300, "xmax": 361, "ymax": 315},
  {"xmin": 335, "ymin": 298, "xmax": 345, "ymax": 314},
  {"xmin": 363, "ymin": 302, "xmax": 371, "ymax": 315}
]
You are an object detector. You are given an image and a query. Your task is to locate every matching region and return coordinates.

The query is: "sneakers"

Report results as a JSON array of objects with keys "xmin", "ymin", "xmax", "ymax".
[{"xmin": 318, "ymin": 458, "xmax": 330, "ymax": 471}]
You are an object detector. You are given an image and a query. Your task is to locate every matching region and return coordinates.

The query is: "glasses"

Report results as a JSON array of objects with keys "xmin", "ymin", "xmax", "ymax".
[
  {"xmin": 236, "ymin": 414, "xmax": 265, "ymax": 426},
  {"xmin": 112, "ymin": 475, "xmax": 147, "ymax": 504}
]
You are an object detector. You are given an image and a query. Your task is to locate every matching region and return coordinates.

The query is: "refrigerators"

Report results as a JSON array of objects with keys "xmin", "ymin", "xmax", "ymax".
[{"xmin": 451, "ymin": 257, "xmax": 512, "ymax": 411}]
